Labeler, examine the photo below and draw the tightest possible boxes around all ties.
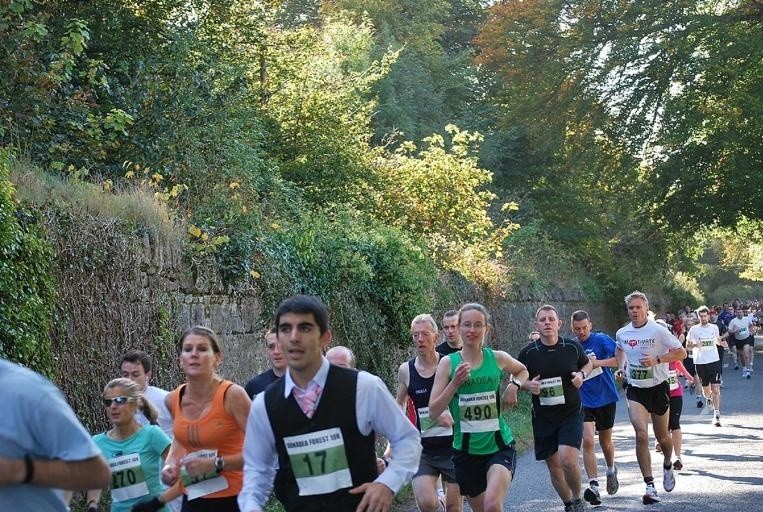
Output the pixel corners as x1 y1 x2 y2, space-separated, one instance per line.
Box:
291 385 322 417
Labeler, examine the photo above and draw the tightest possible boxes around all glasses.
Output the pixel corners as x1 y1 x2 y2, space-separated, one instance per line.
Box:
458 322 488 329
102 395 137 406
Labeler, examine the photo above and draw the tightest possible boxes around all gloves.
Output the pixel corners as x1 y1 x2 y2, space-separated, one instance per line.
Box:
128 496 165 512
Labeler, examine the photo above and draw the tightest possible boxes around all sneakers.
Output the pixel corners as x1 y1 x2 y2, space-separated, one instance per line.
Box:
714 416 721 427
662 462 676 492
573 499 585 512
607 466 619 496
642 485 661 506
673 459 684 471
683 361 757 409
583 486 602 506
563 501 573 512
